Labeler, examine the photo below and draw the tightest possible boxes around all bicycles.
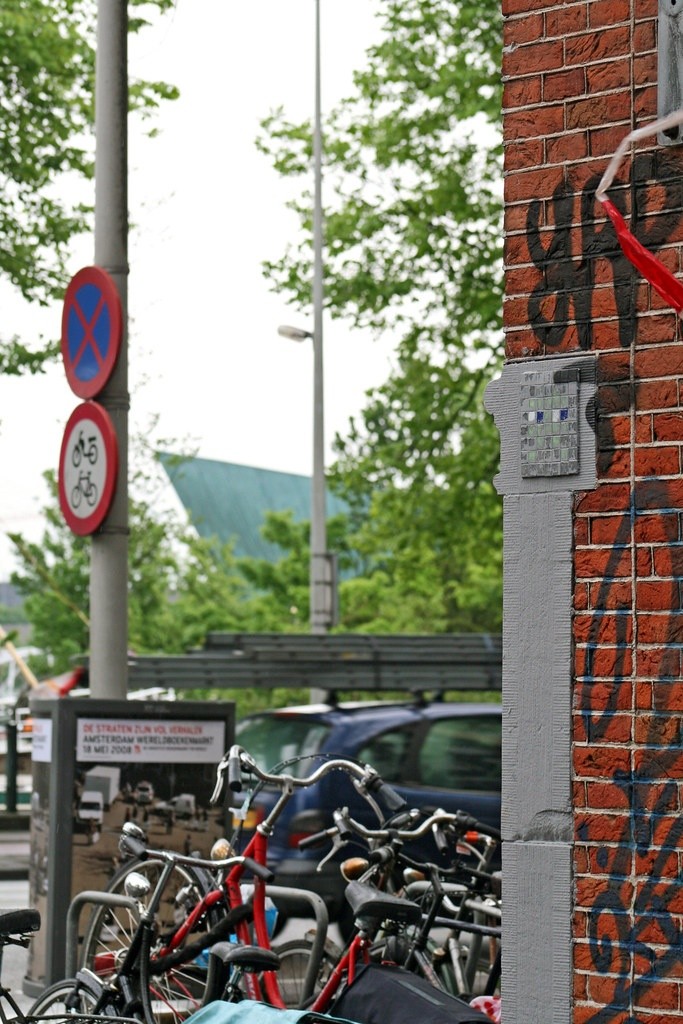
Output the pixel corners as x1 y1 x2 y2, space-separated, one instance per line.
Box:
0 743 501 1024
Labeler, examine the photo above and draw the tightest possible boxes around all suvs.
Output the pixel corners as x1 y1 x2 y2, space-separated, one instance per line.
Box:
235 700 502 904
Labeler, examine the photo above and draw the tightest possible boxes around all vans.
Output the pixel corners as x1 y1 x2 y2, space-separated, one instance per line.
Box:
76 790 103 833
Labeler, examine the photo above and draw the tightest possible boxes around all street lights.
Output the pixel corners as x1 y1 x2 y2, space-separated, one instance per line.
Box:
275 324 339 635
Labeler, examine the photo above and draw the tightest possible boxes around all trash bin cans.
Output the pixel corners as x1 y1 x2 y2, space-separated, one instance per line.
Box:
21 694 236 1000
